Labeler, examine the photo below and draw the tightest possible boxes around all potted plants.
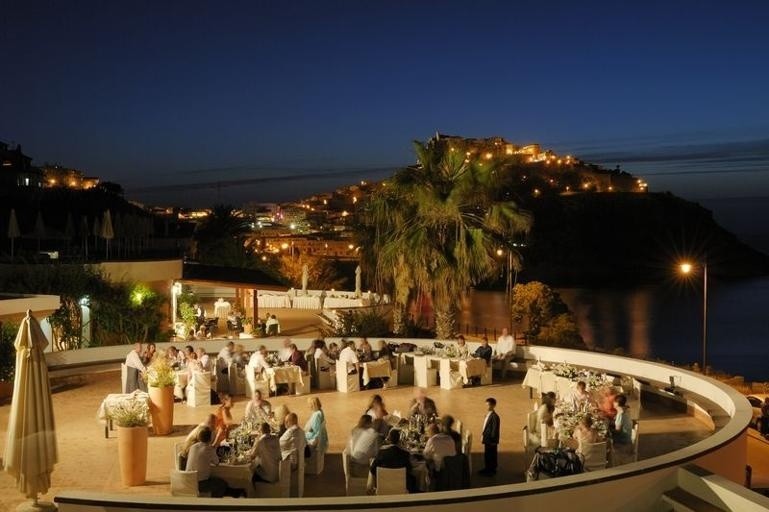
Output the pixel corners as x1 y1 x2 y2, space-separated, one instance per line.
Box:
104 395 155 487
138 354 179 435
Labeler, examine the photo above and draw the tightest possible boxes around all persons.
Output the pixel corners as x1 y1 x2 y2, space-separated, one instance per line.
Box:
124 299 638 499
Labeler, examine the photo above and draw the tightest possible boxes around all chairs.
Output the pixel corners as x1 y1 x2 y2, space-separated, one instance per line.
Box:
176 314 283 340
121 348 493 408
522 364 641 480
342 411 473 495
168 415 327 498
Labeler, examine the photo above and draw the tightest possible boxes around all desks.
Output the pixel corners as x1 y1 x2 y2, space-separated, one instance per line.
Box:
96 393 151 438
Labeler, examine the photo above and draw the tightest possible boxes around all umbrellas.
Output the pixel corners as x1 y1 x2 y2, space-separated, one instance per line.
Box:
0 306 60 506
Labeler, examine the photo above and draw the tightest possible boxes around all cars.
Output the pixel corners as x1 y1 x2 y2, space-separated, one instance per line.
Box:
745 392 769 431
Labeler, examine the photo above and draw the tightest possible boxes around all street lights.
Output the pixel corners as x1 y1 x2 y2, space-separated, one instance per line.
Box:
170 282 182 339
282 241 294 274
678 258 708 372
495 243 515 336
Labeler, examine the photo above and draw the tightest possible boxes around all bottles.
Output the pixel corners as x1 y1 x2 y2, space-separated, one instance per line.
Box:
224 412 255 457
556 393 587 419
564 361 566 366
399 412 439 451
